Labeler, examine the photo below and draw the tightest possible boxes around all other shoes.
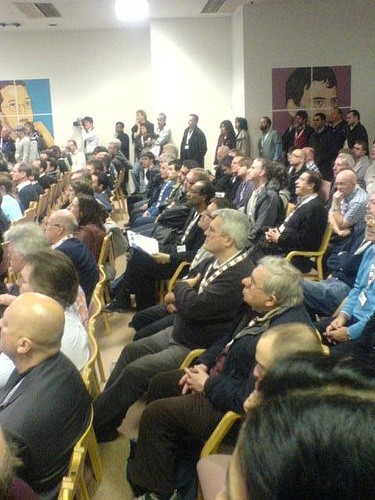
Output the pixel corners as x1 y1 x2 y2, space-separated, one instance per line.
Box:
134 488 180 500
100 292 131 312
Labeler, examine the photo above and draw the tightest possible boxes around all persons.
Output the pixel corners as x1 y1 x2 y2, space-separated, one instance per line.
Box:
135 122 160 157
114 121 130 161
78 116 100 157
286 110 314 150
257 116 283 163
180 113 207 168
14 125 33 164
131 109 155 142
328 106 350 140
344 110 369 155
23 121 45 151
308 113 337 180
0 126 16 163
150 112 172 145
234 116 251 157
1 140 375 500
213 119 237 165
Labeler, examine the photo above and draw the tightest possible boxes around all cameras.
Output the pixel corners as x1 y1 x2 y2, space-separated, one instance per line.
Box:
73 121 83 127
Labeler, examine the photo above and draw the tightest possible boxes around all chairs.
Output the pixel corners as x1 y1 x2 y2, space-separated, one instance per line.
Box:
85 289 100 339
94 263 111 337
66 401 105 500
159 174 339 460
2 166 127 262
81 330 102 408
94 229 115 305
